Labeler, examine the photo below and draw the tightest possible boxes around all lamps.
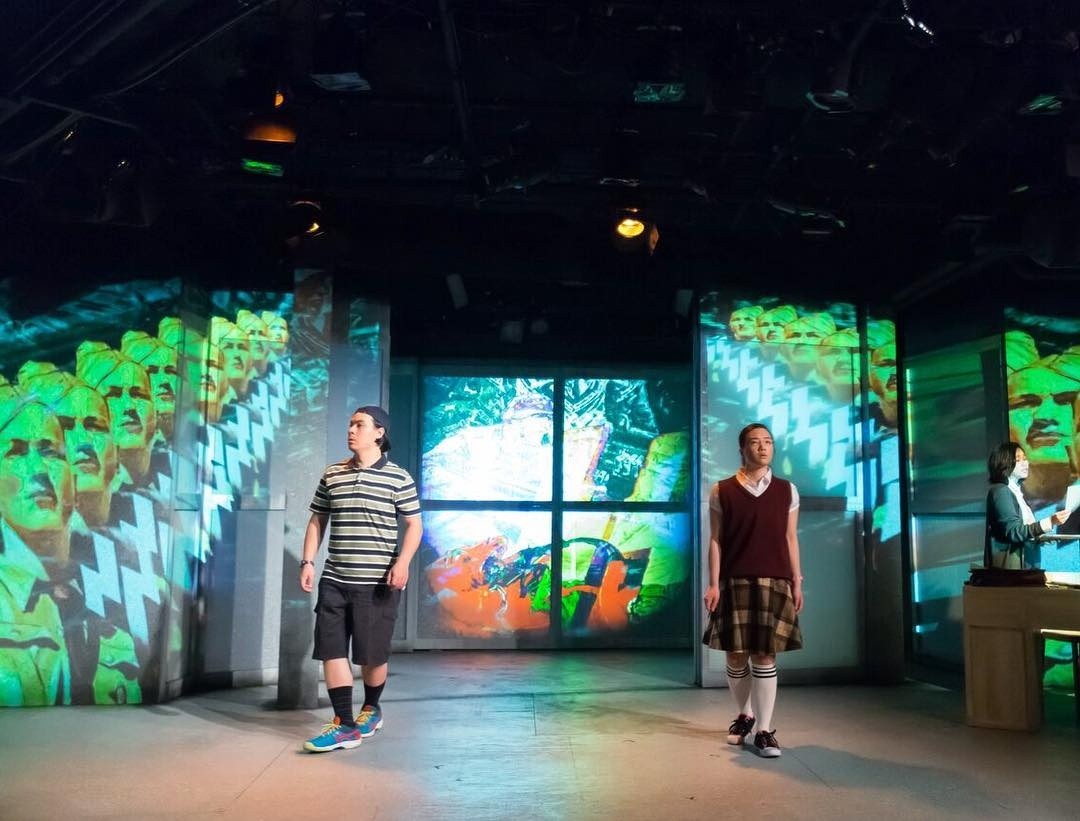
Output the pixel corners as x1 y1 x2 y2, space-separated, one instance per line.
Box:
616 206 645 241
286 200 328 241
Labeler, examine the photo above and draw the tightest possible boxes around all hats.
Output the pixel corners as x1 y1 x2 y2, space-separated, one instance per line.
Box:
357 405 393 453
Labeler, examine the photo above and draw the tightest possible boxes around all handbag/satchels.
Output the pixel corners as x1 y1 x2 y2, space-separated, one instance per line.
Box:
964 568 1050 589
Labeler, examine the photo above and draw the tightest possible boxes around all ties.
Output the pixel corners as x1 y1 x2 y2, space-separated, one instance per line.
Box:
35 580 94 706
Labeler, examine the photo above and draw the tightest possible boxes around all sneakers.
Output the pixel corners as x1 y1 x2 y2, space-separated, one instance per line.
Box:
302 714 362 753
753 731 781 757
353 703 383 737
727 712 757 745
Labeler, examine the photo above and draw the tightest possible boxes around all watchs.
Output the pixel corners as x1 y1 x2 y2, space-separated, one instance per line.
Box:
299 560 314 568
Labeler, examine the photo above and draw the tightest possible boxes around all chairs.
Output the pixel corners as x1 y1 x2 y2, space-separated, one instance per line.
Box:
1042 570 1080 719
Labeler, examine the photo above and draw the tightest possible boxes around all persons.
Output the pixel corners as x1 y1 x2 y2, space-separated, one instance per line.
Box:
696 295 1080 501
983 443 1073 570
1 310 288 708
704 423 804 756
298 406 425 752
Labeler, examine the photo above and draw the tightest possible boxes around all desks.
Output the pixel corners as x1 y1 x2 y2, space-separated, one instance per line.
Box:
963 582 1080 734
